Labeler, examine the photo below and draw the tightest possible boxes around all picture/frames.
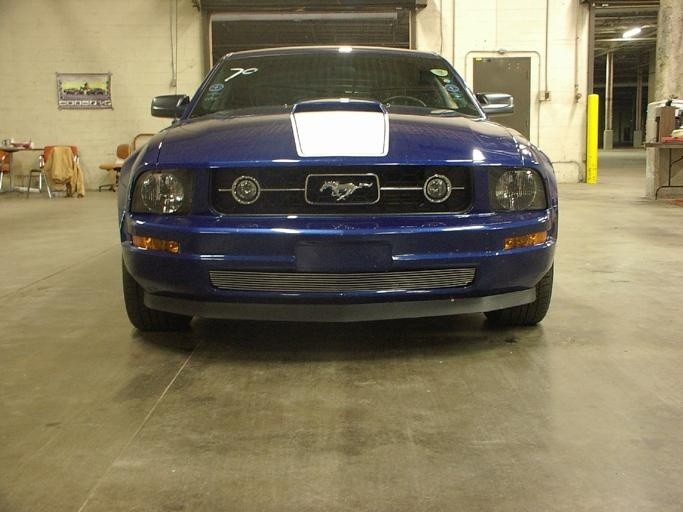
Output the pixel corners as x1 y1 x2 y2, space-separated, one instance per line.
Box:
55 71 113 110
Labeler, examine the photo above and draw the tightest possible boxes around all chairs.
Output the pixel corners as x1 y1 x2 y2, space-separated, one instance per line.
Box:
97 142 129 192
28 144 77 199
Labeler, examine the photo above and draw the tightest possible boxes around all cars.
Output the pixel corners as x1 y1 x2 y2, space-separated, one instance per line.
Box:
117 43 557 333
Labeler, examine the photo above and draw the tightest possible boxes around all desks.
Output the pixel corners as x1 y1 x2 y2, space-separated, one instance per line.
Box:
642 139 683 201
0 142 43 194
